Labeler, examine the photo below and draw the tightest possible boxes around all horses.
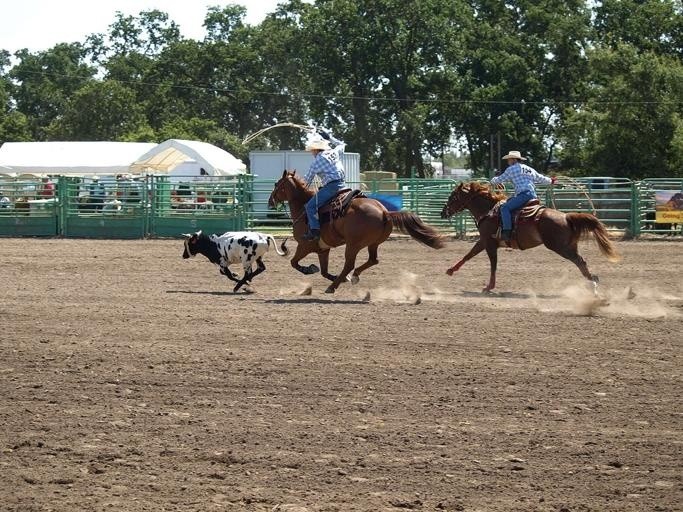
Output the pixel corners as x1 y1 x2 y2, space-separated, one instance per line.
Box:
440 181 616 299
267 169 446 293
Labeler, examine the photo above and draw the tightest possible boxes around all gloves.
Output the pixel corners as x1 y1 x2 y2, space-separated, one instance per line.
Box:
551 176 557 184
318 129 330 140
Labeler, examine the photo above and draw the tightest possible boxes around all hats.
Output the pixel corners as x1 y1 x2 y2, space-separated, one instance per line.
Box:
502 151 527 160
308 140 331 150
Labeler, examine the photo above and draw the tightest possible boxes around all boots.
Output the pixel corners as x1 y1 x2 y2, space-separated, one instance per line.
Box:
300 229 320 241
500 230 512 240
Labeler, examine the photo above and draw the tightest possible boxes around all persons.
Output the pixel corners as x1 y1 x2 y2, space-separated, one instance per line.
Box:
485 150 557 242
299 126 346 241
0 175 230 213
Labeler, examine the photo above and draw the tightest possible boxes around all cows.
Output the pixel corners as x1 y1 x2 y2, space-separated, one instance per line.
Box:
179 230 292 295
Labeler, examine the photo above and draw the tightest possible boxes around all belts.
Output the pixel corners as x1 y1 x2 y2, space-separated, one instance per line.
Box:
330 180 344 183
